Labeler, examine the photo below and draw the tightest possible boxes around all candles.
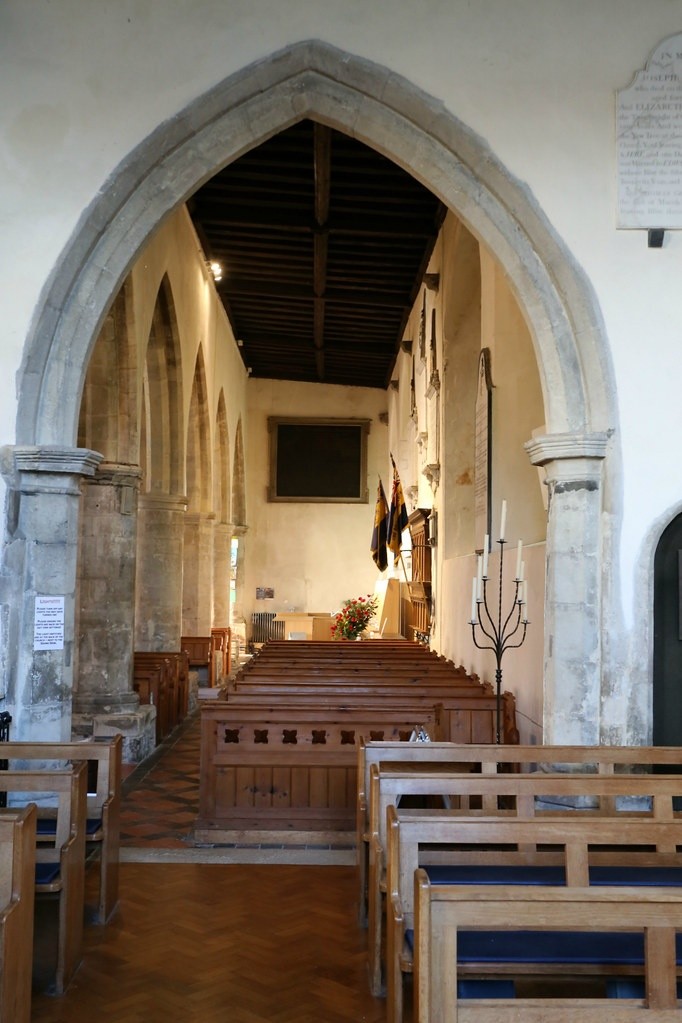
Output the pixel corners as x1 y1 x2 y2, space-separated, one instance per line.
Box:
483 532 490 576
516 538 522 578
477 556 481 602
499 498 507 539
518 561 525 600
523 580 528 620
472 575 477 621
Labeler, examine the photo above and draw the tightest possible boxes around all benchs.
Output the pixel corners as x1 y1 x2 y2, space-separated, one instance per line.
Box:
1 628 682 1023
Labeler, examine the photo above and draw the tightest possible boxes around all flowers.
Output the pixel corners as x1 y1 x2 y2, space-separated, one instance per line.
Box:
330 593 378 641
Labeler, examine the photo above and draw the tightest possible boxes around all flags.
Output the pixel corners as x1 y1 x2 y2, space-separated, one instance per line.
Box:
386 460 411 567
370 479 390 572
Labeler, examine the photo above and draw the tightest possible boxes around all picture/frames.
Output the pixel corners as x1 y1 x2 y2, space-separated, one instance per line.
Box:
266 415 370 505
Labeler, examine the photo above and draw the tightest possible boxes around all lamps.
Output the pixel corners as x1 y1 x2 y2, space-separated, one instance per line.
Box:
206 260 222 282
237 340 243 346
247 367 252 375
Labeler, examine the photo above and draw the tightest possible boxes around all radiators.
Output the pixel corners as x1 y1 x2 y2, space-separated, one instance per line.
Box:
252 613 286 640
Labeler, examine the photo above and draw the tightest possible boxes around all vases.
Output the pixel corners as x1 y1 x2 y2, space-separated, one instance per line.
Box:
348 622 364 642
